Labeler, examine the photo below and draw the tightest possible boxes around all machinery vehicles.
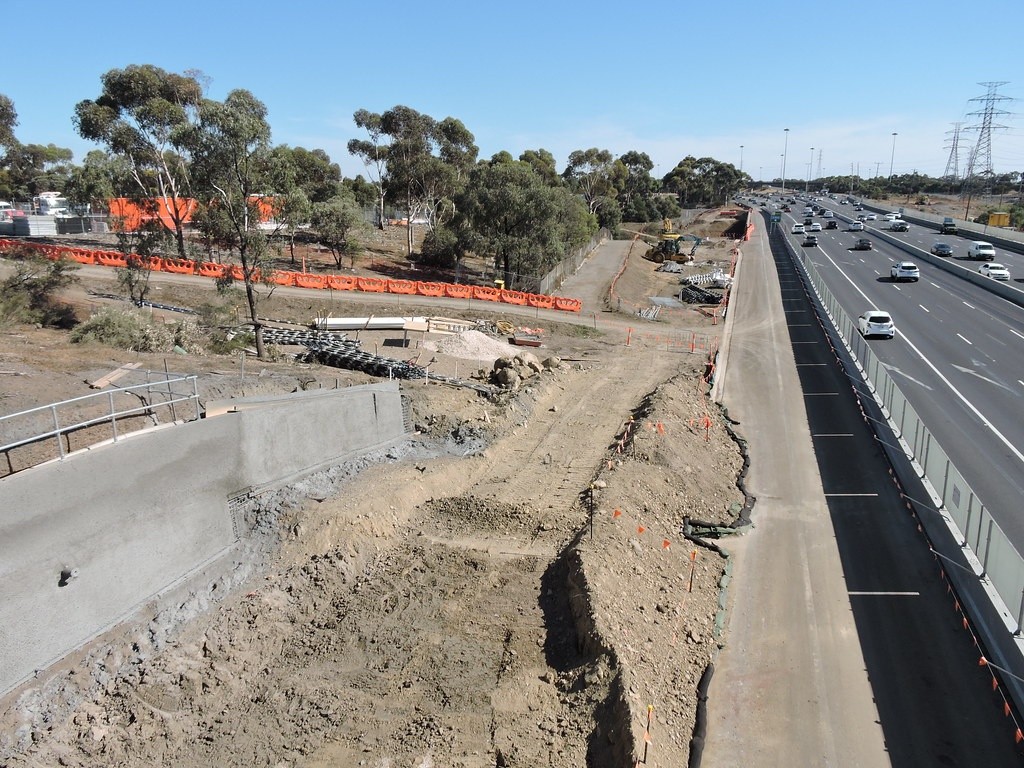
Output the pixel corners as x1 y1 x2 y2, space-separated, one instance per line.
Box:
645 217 702 265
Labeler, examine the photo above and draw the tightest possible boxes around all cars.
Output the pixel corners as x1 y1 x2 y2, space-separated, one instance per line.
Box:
931 242 953 257
855 239 872 250
858 310 895 339
979 262 1011 281
738 191 911 235
802 235 818 247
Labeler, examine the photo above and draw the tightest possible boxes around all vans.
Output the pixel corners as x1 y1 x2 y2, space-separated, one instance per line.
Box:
0 201 15 212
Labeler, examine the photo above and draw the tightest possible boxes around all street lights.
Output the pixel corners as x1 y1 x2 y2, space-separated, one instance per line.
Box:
889 132 898 184
805 163 812 197
740 145 743 173
782 129 790 192
809 148 815 181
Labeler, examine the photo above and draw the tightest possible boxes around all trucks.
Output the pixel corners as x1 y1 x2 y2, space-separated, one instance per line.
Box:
32 191 70 216
968 240 995 261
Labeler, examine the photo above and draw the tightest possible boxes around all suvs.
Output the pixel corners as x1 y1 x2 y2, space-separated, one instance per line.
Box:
891 262 919 282
941 222 959 235
